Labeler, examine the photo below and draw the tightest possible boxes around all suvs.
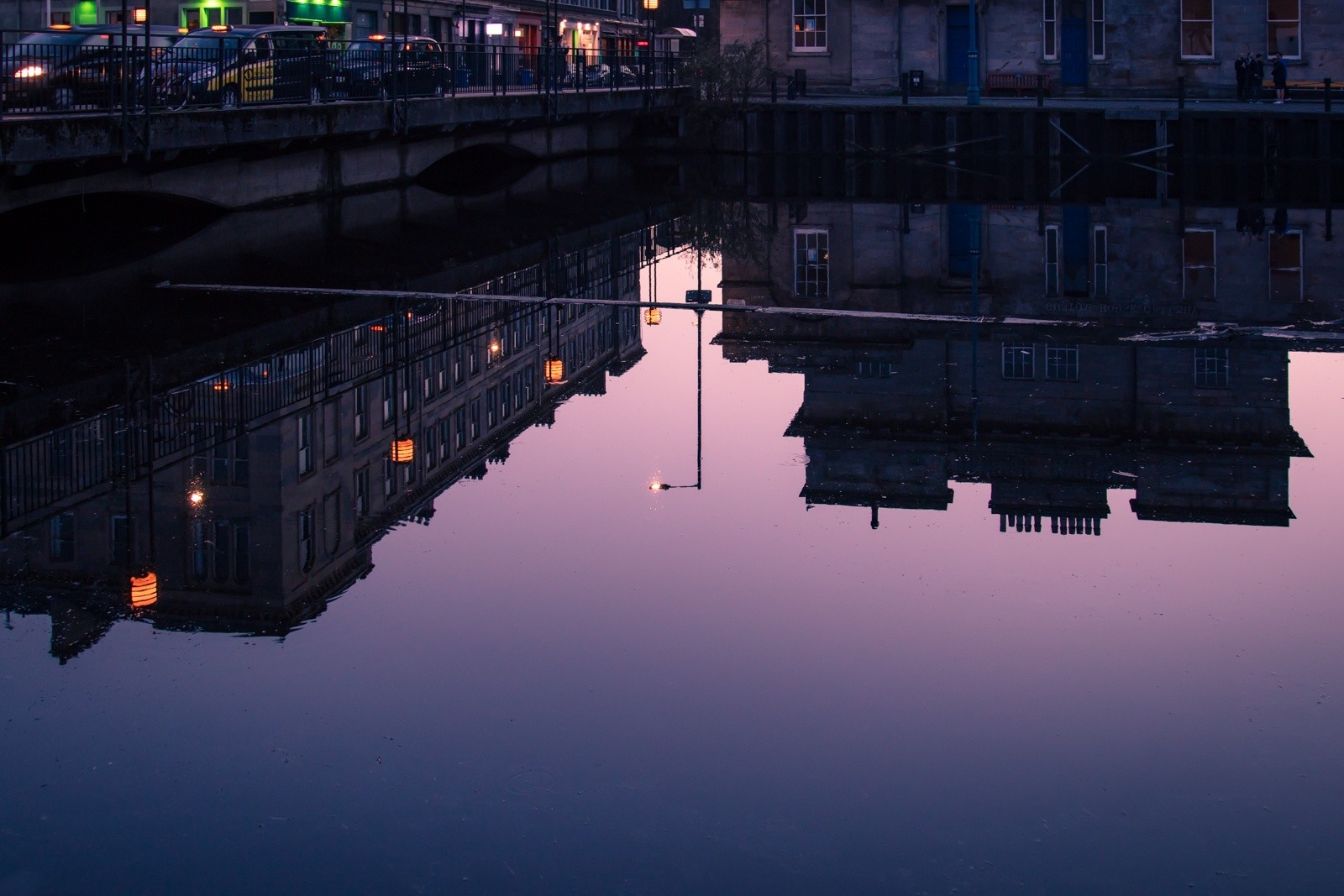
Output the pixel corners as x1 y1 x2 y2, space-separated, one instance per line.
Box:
0 24 190 110
329 33 448 99
135 24 329 109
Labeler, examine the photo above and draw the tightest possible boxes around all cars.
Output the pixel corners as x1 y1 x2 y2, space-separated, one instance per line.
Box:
572 64 676 88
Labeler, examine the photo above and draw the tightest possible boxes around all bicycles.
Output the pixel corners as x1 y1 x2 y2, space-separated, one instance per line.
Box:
125 48 193 115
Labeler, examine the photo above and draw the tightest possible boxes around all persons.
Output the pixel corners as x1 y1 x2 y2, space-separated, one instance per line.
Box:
1271 52 1292 104
538 36 568 93
307 32 336 103
416 41 435 60
1234 52 1265 104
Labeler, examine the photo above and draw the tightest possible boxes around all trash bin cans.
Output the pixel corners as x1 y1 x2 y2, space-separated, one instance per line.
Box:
458 69 471 87
522 69 535 86
794 68 806 97
909 70 923 97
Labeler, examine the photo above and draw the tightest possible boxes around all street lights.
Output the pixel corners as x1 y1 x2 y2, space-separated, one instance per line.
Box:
648 248 705 489
